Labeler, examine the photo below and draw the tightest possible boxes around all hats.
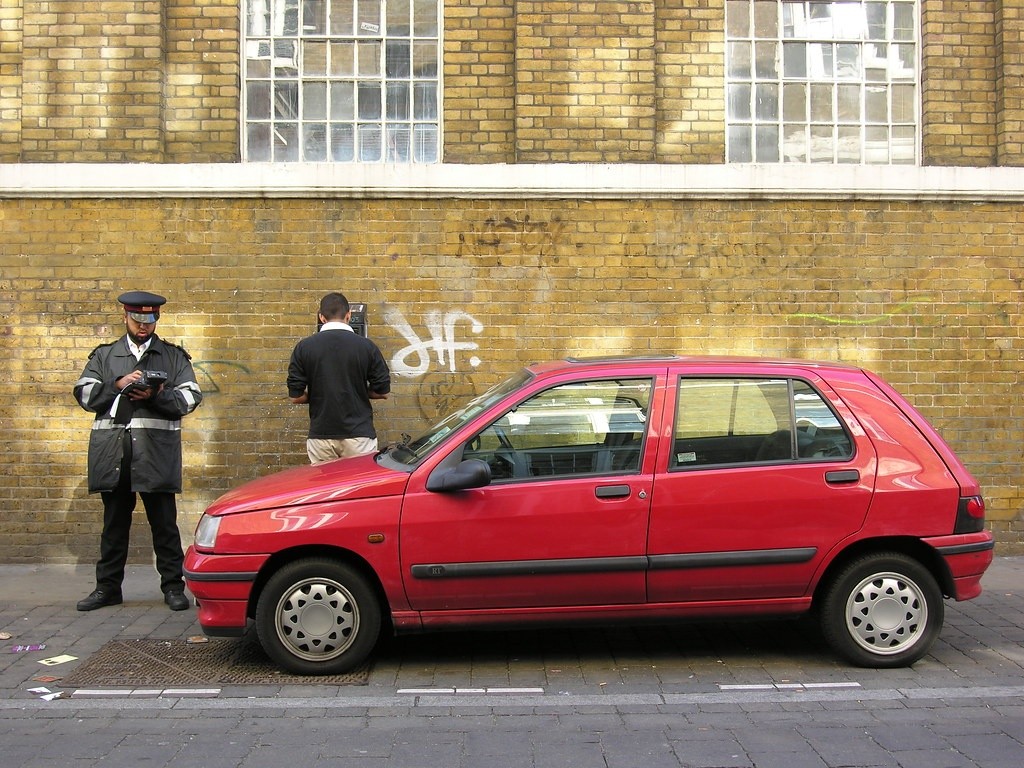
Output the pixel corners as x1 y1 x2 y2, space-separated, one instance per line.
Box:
117 290 168 325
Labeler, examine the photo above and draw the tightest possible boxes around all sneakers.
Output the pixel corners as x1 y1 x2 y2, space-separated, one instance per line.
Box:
163 588 189 611
76 588 123 611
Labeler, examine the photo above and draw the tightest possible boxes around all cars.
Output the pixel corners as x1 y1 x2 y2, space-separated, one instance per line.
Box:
181 354 996 677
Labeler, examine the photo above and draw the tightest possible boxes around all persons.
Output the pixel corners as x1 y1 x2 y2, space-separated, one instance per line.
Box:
73 291 203 610
287 293 391 464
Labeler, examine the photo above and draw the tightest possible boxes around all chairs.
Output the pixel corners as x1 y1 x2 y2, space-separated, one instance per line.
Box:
594 405 636 472
754 429 818 460
803 436 851 457
612 436 678 470
